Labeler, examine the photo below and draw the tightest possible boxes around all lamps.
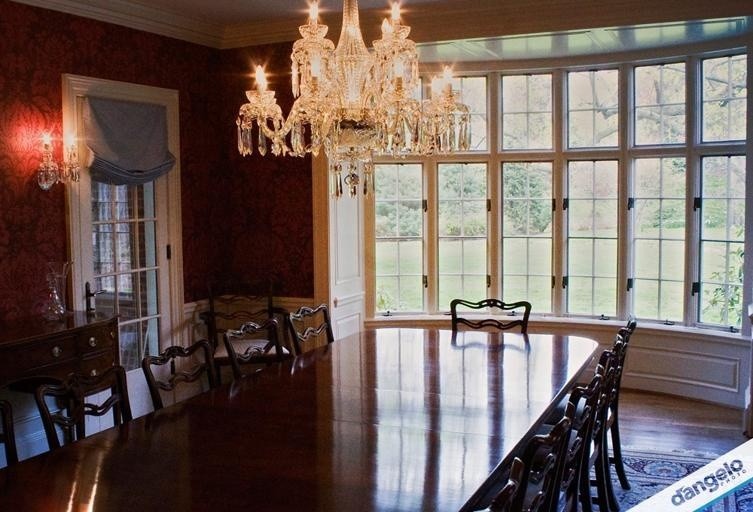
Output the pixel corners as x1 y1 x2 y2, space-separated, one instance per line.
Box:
233 0 469 198
37 132 81 189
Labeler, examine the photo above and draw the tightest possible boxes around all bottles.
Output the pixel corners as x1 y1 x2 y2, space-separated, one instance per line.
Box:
40 284 65 322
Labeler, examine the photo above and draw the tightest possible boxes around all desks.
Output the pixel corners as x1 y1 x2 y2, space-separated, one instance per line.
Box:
4 323 601 509
0 308 124 427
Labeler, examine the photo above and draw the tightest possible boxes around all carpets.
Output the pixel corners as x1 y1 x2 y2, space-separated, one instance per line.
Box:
563 440 753 512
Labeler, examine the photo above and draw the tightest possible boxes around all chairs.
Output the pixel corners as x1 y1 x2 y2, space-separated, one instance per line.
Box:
222 318 281 376
287 304 334 354
140 339 217 411
0 397 17 467
472 315 637 510
33 364 133 449
449 297 531 332
